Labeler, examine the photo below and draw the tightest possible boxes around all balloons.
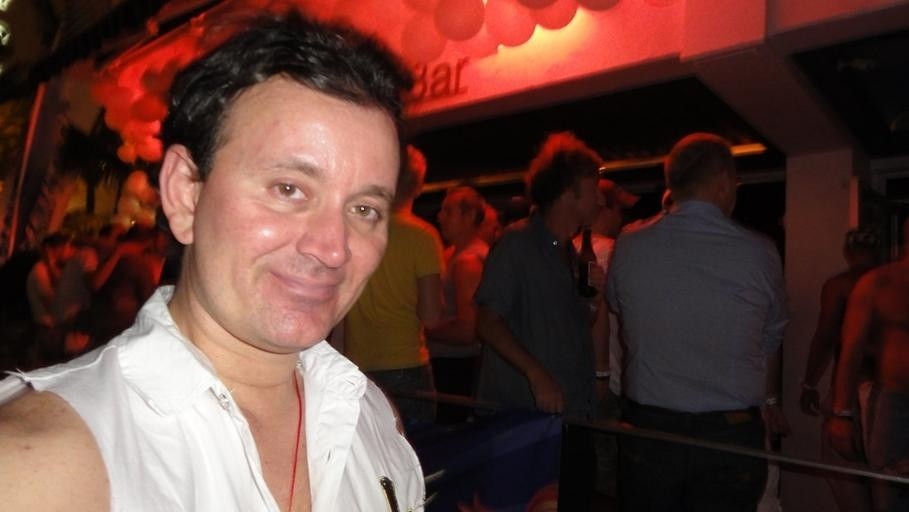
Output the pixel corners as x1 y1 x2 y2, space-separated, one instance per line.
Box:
55 2 623 237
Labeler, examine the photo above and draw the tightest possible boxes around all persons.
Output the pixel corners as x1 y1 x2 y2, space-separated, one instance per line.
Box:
832 220 907 510
799 228 883 510
1 123 793 511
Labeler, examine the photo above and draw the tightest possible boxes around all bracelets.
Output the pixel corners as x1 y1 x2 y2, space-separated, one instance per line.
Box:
801 383 819 392
832 407 856 419
